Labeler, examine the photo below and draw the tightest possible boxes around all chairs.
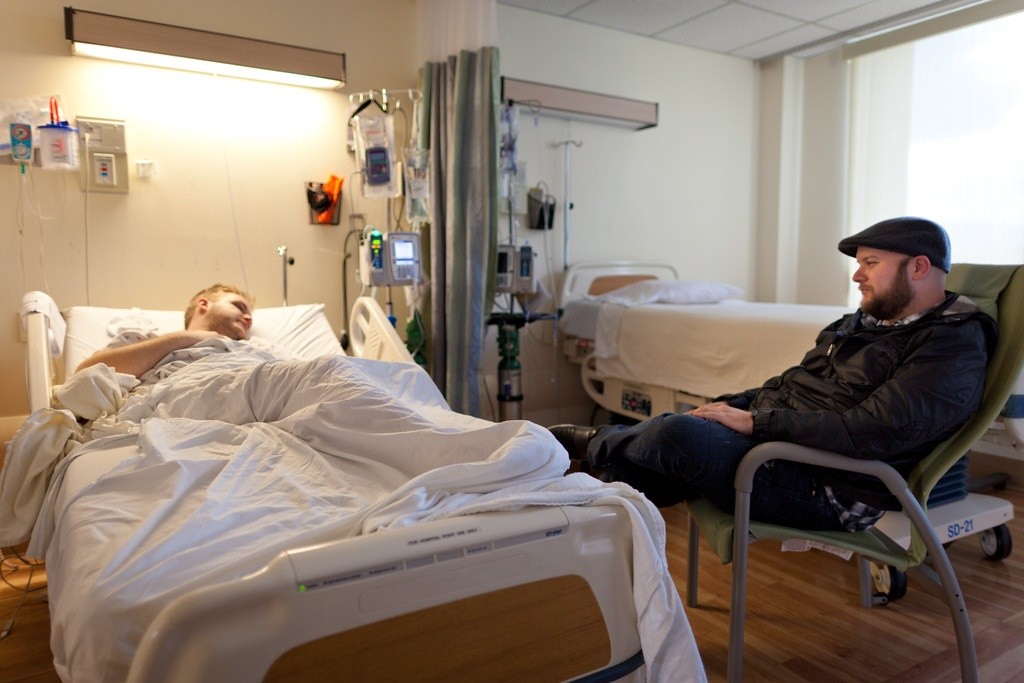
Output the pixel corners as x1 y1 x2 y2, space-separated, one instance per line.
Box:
685 264 1024 683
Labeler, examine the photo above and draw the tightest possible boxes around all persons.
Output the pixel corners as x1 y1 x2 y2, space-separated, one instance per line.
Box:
545 216 1003 533
72 282 257 377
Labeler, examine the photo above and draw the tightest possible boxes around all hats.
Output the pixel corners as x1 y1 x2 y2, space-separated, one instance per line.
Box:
837 217 951 274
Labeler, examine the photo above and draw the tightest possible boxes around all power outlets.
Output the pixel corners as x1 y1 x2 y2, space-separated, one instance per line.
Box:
136 161 155 178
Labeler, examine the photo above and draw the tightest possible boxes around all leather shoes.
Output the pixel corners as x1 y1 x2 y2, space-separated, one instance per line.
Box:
546 424 600 459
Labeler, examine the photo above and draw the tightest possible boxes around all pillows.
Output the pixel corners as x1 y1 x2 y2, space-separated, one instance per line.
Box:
624 279 725 304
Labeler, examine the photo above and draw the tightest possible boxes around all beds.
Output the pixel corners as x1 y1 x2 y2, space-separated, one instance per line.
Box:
19 291 668 683
558 260 1024 599
